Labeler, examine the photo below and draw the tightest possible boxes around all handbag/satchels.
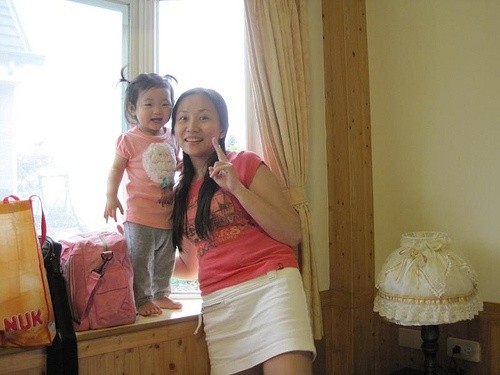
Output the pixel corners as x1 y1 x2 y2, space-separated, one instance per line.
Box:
0 195 56 347
59 225 136 332
38 235 62 277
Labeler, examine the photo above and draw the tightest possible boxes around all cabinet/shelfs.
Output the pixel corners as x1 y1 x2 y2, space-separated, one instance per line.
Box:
0 318 212 375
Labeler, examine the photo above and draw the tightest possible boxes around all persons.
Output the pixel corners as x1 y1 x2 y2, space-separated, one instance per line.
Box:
104 65 183 317
168 88 318 375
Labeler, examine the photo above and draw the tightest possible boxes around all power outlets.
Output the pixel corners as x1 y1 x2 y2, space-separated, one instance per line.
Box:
447 337 481 363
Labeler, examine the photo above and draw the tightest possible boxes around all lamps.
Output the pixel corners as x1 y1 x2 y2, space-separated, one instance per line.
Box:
372 230 483 375
